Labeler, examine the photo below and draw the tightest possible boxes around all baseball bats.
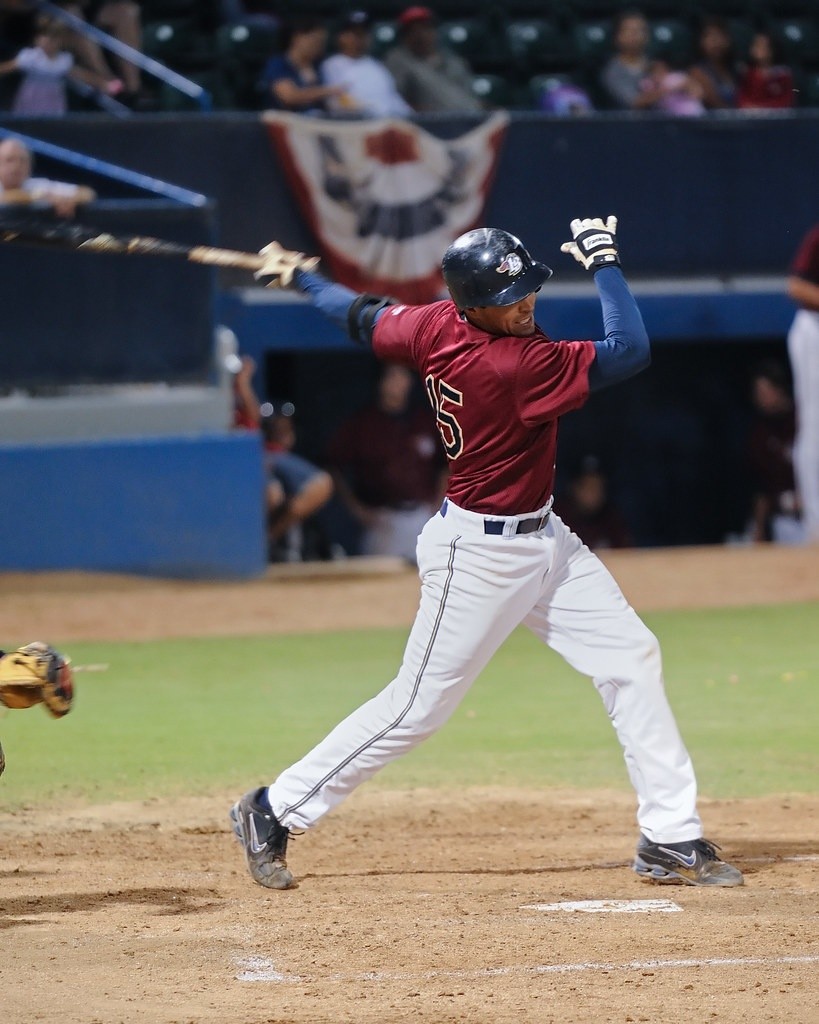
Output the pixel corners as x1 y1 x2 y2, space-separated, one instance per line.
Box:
0 220 329 274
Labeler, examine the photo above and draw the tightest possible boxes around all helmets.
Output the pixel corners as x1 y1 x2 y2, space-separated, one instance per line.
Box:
440 227 554 308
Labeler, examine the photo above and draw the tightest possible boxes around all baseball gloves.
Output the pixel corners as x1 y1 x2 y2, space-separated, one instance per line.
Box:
0 643 73 718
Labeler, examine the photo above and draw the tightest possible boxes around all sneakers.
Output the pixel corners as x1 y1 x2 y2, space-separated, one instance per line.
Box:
632 833 745 888
228 786 307 889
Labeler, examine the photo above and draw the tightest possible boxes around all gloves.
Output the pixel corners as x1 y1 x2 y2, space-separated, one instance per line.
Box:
251 241 322 291
562 215 622 275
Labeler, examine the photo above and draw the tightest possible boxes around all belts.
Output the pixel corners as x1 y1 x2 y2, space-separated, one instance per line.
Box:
438 502 551 536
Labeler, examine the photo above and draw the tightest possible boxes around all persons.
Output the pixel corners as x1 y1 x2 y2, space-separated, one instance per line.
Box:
234 356 452 571
0 642 73 778
0 0 144 118
742 366 802 543
0 137 97 220
233 216 748 891
785 224 819 540
250 8 796 117
551 463 637 551
